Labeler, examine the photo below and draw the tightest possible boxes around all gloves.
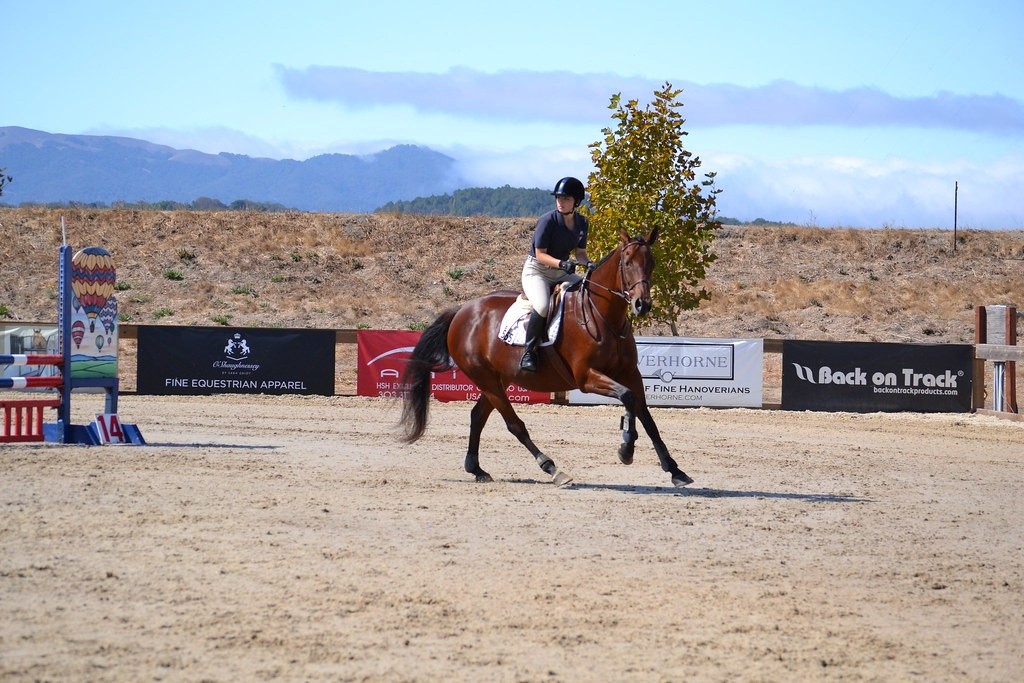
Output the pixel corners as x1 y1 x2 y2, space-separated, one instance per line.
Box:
558 260 576 275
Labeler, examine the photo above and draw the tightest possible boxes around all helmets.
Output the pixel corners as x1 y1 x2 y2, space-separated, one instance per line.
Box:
550 177 585 200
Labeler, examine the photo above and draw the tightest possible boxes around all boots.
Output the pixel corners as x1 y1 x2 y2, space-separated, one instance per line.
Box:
518 309 548 370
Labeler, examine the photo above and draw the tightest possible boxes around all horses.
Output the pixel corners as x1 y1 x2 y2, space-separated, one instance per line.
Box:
380 219 694 490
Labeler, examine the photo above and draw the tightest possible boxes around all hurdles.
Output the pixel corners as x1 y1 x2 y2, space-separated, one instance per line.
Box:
1 243 148 446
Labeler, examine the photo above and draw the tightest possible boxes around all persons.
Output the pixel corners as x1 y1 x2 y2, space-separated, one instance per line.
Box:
520 177 591 368
